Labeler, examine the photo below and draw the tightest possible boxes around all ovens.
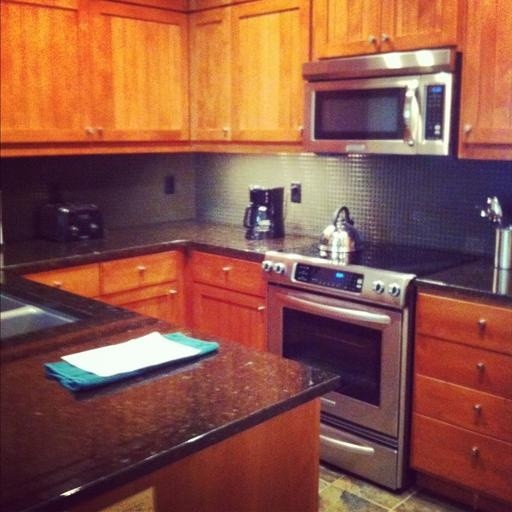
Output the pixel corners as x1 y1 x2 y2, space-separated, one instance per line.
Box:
265 283 411 494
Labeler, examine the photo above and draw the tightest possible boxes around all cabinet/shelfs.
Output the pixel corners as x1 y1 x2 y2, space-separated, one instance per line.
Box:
2 0 192 155
20 249 187 329
456 1 512 164
313 0 463 60
186 246 270 354
190 0 312 157
407 275 510 511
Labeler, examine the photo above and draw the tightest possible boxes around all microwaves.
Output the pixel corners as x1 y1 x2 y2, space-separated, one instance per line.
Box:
301 73 458 157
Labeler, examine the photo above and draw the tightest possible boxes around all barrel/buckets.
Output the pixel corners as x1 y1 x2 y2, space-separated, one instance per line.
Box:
492 224 512 270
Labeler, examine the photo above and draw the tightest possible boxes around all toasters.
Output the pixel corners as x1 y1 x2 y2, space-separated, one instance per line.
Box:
37 203 105 241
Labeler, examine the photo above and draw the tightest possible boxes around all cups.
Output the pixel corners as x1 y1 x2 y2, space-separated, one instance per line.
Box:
494 227 512 270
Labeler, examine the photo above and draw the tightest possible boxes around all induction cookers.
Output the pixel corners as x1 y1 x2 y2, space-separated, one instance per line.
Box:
261 241 481 309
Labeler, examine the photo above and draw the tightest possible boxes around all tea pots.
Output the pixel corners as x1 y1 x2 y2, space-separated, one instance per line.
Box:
314 205 365 252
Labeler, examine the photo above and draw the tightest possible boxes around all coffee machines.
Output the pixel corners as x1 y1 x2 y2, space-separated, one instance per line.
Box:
242 184 285 239
